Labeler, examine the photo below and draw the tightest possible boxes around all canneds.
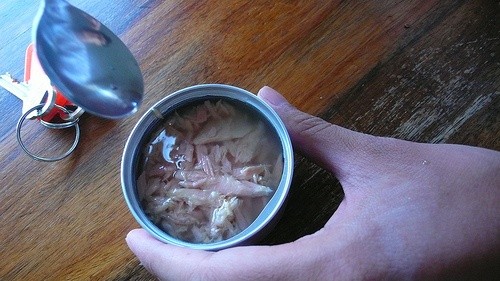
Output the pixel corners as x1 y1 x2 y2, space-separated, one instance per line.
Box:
121 83 295 251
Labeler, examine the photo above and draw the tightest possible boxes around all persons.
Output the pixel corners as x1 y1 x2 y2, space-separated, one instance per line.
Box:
123 84 500 280
